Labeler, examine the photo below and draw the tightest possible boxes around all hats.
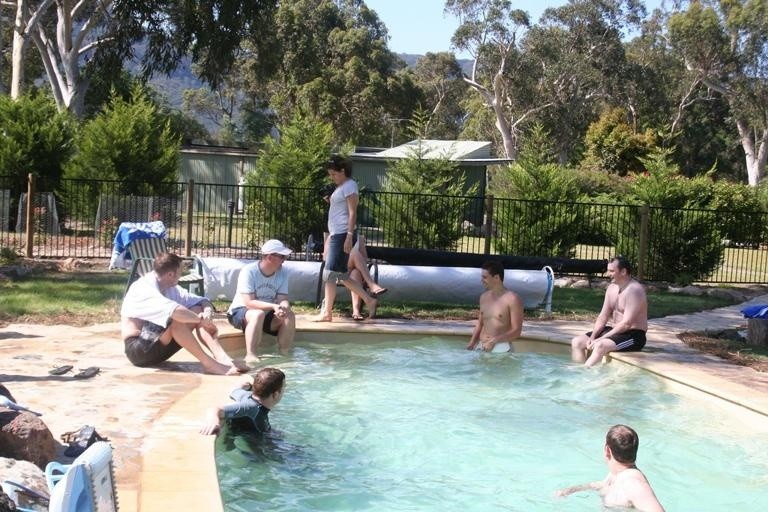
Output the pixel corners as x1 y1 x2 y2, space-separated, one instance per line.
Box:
261 239 293 256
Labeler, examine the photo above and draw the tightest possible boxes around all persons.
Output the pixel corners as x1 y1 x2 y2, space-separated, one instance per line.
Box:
553 424 666 512
570 257 648 367
120 252 250 377
323 229 388 321
465 259 524 352
307 156 379 326
198 367 314 479
225 239 296 363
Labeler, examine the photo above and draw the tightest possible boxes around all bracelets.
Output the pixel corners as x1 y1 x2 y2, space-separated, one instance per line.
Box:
347 231 353 234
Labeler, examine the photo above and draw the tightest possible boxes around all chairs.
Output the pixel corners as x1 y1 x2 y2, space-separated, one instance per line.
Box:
119 221 204 301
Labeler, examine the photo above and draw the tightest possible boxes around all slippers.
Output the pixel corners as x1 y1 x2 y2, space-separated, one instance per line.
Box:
351 314 364 321
372 288 388 296
